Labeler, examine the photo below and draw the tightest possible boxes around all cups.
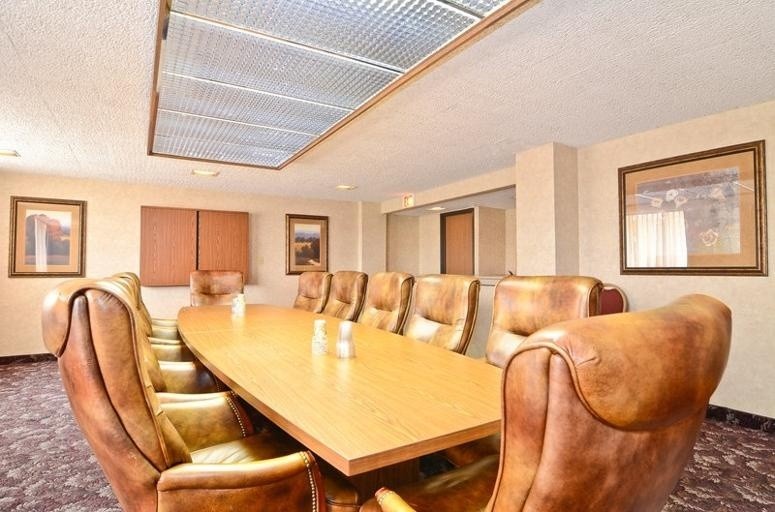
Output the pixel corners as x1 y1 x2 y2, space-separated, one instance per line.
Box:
310 319 329 357
335 320 356 359
232 293 246 314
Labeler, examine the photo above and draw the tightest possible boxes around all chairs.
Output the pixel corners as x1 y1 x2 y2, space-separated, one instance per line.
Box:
40 271 358 512
357 271 415 335
320 272 368 322
482 274 629 370
402 273 480 356
357 293 732 512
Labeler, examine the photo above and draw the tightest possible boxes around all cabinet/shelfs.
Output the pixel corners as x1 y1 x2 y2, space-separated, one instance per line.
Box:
139 205 250 288
440 208 475 276
176 304 503 477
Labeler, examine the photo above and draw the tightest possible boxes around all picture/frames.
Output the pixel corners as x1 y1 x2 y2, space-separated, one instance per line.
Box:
7 195 88 278
616 139 769 277
284 213 329 276
